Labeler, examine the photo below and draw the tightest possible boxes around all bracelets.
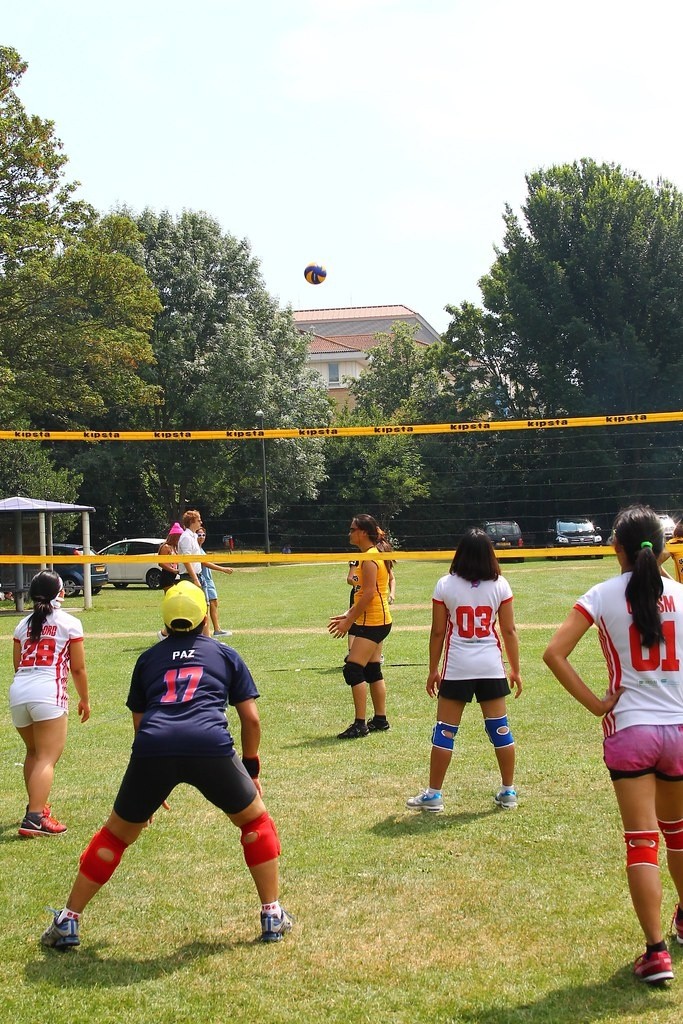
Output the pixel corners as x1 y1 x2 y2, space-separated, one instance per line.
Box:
242 755 261 779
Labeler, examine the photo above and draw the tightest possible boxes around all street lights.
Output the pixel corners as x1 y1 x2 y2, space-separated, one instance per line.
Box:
254 408 271 554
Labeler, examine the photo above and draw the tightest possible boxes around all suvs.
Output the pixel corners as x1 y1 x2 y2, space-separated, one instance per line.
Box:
47 543 110 598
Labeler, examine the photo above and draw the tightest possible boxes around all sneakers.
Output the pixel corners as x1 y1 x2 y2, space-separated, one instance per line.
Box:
670 903 683 944
26 804 52 817
633 940 674 983
405 790 444 813
260 908 295 943
337 723 371 739
366 717 389 732
494 790 516 809
18 813 68 837
39 908 80 948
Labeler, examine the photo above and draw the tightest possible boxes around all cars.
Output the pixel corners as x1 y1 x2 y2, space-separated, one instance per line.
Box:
97 537 166 591
658 515 677 541
548 518 604 561
479 519 525 563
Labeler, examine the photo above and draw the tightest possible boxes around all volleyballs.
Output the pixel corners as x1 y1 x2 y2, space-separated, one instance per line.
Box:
304 262 327 285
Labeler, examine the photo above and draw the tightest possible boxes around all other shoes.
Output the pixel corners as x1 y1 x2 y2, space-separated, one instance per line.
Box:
157 630 167 642
213 630 232 637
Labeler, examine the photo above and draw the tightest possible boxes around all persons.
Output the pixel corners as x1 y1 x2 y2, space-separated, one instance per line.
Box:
543 505 683 984
157 510 233 638
41 584 294 948
403 528 523 814
325 513 397 740
7 570 90 839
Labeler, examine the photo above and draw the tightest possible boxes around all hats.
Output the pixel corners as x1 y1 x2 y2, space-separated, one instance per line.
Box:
169 522 184 534
161 580 208 632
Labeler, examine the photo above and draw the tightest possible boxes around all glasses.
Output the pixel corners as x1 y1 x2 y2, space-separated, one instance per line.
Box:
349 528 360 534
197 519 201 522
197 533 206 538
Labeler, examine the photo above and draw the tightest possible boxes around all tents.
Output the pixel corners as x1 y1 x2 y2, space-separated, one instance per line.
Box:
0 495 96 609
